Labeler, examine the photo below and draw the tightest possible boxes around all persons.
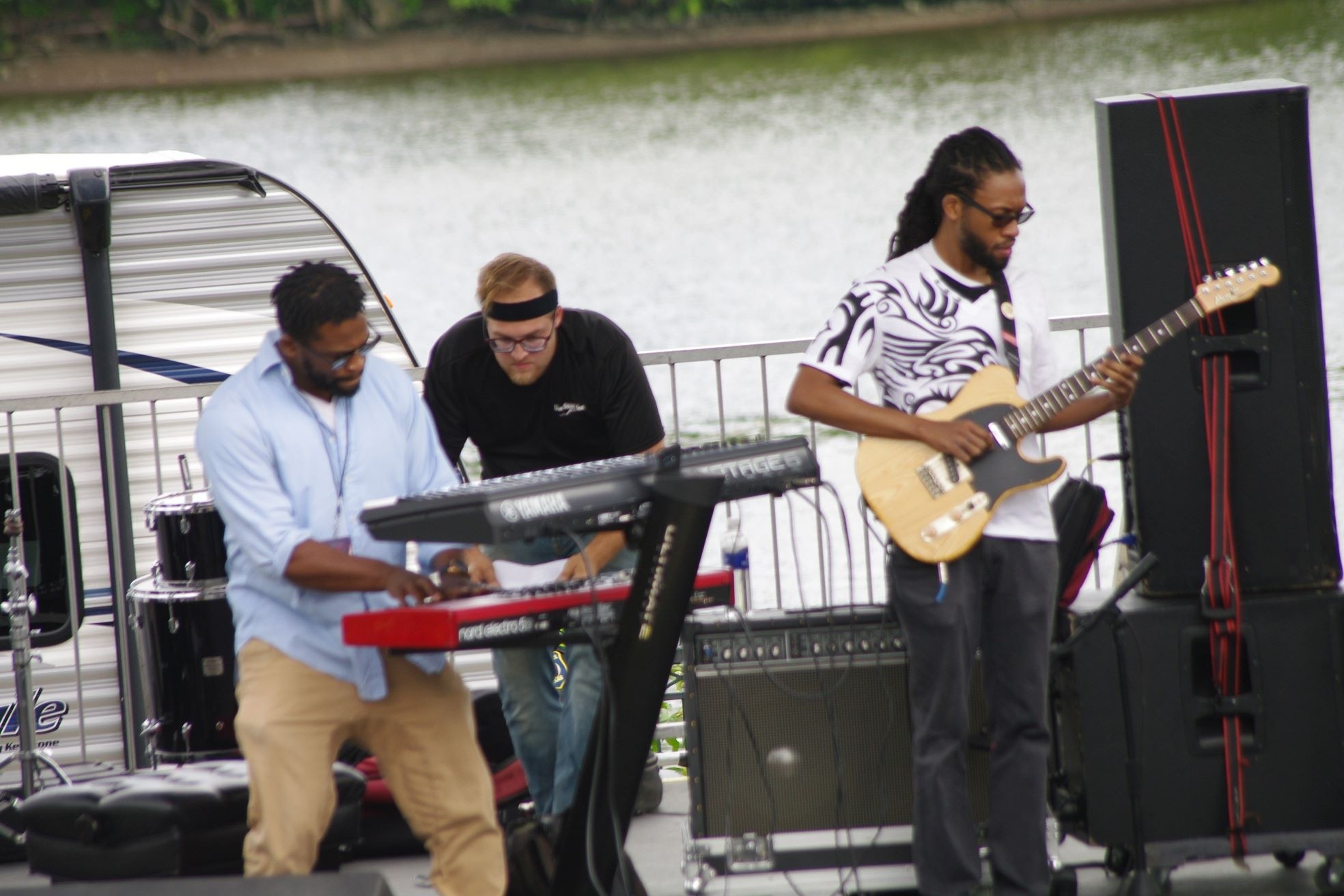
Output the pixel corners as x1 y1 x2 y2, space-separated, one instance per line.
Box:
786 124 1144 896
423 250 666 825
194 260 510 894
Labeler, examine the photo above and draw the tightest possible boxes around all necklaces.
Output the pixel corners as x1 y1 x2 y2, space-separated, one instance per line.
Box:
307 394 352 517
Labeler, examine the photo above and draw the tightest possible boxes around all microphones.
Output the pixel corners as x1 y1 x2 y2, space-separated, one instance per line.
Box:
1075 548 1160 637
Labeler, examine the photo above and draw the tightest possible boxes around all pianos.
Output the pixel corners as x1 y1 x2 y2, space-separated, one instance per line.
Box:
339 562 738 896
356 429 827 548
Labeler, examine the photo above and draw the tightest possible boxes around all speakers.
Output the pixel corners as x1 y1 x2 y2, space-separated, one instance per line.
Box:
1069 588 1344 860
685 618 914 846
1094 79 1344 591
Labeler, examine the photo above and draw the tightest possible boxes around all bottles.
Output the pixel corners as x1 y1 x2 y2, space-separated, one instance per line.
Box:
720 516 752 619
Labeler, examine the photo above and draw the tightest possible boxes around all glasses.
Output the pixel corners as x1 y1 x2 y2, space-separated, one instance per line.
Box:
483 307 556 353
952 191 1036 228
300 321 382 373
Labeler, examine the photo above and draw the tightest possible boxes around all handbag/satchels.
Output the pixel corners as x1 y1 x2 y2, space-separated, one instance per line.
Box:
1049 477 1116 609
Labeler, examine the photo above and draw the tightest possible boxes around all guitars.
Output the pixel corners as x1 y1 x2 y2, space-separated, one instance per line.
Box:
852 257 1282 566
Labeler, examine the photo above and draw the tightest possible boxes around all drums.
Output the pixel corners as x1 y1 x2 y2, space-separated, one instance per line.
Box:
142 485 230 590
124 569 245 766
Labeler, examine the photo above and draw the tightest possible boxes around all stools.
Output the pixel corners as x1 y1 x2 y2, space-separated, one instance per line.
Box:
12 759 368 886
